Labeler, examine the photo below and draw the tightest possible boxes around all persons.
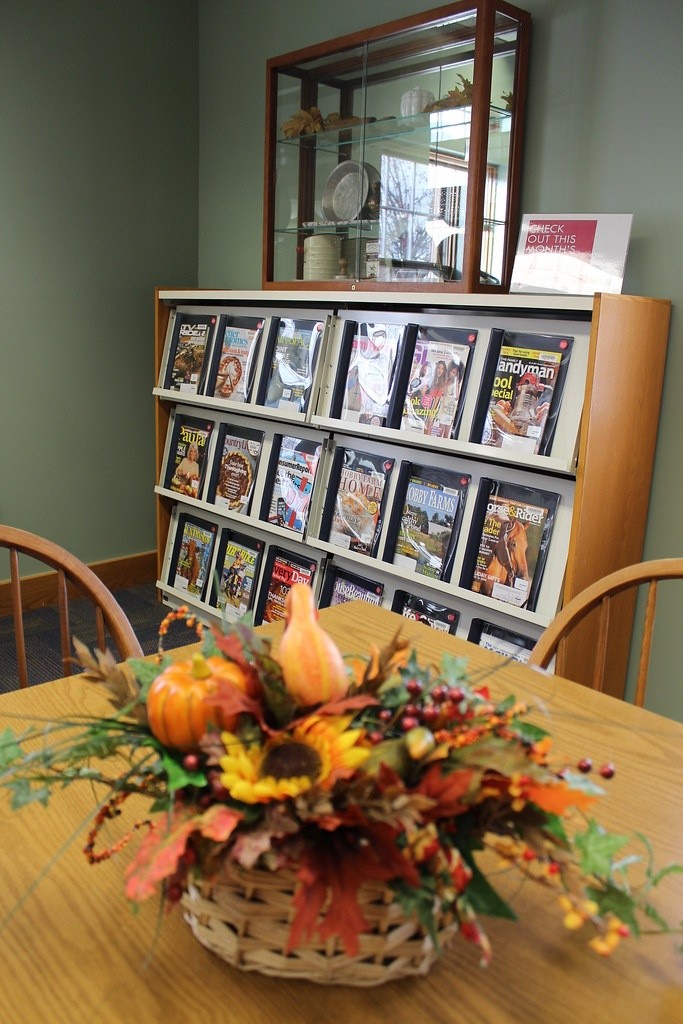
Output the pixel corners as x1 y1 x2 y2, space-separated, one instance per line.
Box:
422 359 465 439
224 550 247 597
173 440 200 487
516 379 533 396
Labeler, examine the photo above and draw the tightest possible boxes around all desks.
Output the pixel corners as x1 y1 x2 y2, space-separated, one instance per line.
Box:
0 598 683 1024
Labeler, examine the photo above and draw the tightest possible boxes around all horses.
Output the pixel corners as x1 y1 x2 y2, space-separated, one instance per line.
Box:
472 510 531 597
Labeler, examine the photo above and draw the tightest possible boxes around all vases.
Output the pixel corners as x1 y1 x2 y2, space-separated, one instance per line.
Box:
183 835 463 983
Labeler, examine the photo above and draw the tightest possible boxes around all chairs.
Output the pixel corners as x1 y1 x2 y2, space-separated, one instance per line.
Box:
528 557 683 708
0 524 146 688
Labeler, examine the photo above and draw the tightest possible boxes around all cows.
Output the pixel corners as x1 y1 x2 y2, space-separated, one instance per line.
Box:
180 540 200 584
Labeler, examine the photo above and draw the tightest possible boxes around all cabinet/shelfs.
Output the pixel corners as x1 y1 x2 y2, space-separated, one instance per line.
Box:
262 0 532 294
153 287 672 699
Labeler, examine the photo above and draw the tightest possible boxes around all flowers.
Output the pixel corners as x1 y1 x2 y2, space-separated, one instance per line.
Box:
0 583 683 974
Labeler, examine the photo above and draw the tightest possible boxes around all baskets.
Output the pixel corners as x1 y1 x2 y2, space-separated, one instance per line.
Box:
180 850 459 987
